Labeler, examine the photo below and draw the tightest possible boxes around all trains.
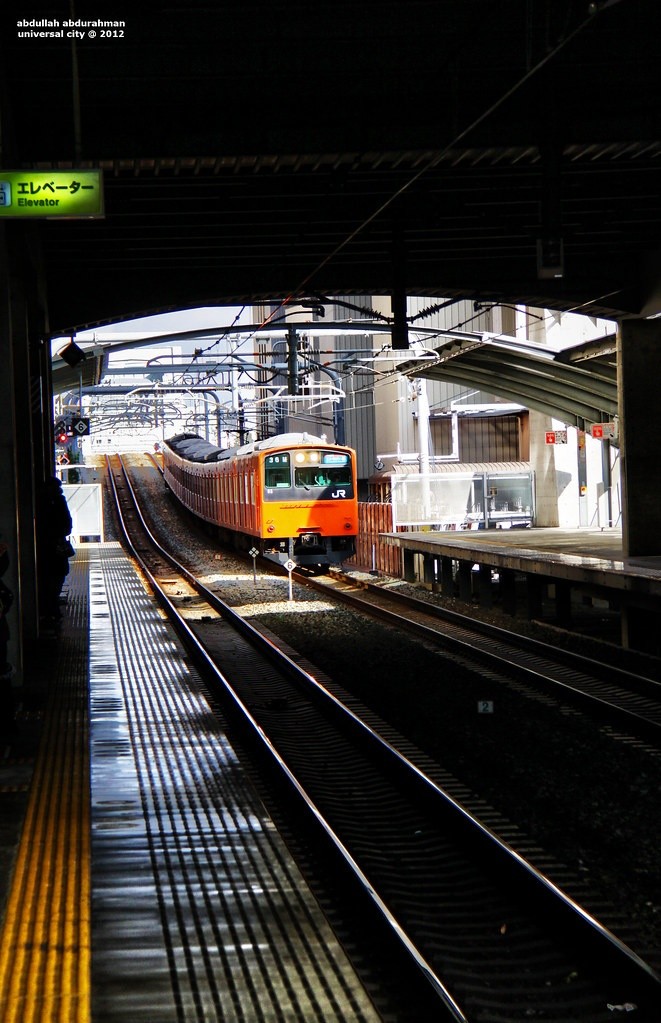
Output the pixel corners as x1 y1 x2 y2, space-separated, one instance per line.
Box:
162 432 358 575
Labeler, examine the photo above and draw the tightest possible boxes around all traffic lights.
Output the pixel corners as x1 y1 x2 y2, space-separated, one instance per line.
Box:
57 421 67 444
